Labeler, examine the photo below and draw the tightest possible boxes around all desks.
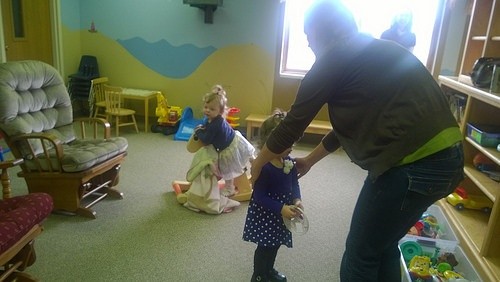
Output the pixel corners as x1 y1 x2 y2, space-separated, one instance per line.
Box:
122 87 160 134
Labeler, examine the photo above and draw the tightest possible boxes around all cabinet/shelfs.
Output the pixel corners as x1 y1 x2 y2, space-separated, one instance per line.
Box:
430 0 500 282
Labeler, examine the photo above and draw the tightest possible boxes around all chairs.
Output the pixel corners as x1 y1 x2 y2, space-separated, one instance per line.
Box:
67 55 102 118
105 91 139 137
0 157 54 282
0 60 130 219
92 77 119 126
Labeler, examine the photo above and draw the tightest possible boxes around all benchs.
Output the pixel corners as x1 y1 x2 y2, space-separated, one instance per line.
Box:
246 114 332 143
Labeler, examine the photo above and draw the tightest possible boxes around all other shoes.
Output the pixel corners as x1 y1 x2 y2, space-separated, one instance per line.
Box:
251 273 274 282
270 269 287 282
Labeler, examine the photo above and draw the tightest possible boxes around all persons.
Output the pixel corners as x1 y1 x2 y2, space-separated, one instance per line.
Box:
195 92 256 196
243 113 305 282
380 9 416 52
251 0 465 282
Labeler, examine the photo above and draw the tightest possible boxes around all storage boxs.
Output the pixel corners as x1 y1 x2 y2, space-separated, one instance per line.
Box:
465 122 500 147
398 238 484 282
399 204 460 251
